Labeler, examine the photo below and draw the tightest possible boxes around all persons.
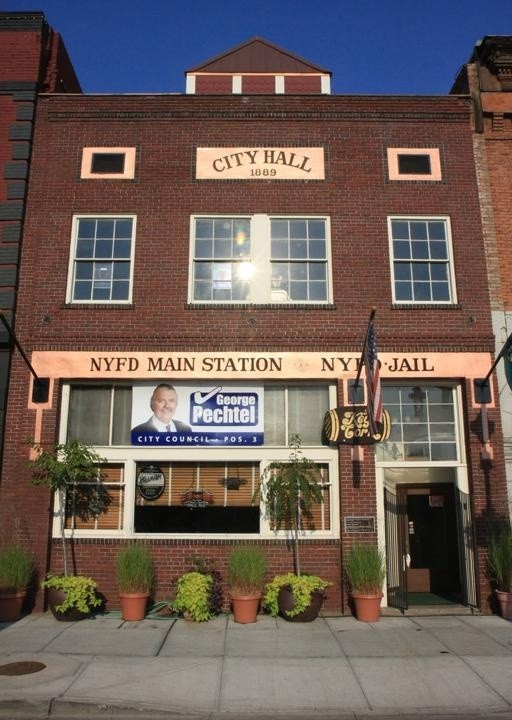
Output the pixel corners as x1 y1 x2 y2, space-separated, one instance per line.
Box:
129 383 194 436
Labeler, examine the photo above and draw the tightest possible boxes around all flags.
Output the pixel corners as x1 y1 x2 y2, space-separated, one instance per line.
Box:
362 318 385 438
499 332 511 392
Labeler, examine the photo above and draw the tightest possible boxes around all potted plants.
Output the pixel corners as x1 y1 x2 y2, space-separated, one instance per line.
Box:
474 510 512 619
